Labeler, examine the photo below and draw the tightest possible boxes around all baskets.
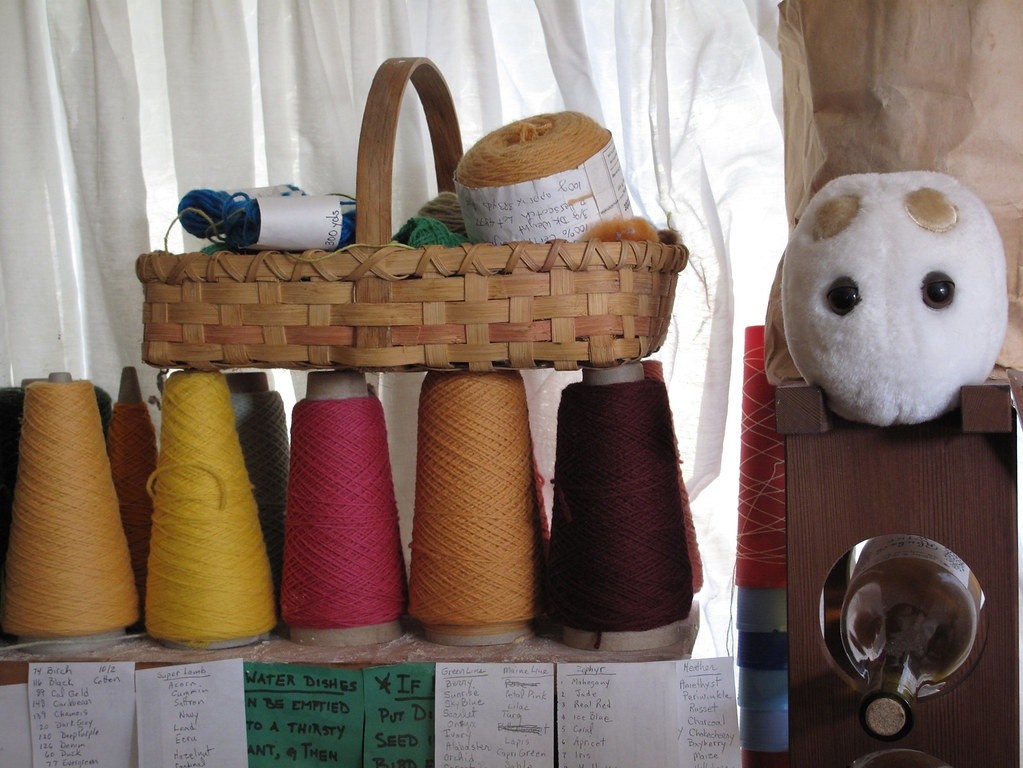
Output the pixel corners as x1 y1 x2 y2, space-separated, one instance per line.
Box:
137 57 690 375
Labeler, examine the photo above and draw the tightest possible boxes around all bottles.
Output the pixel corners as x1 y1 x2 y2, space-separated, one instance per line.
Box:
851 749 952 768
839 533 982 743
